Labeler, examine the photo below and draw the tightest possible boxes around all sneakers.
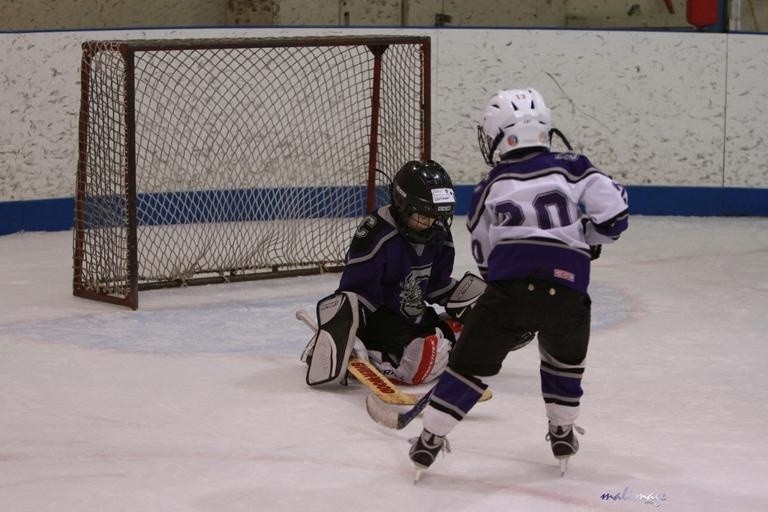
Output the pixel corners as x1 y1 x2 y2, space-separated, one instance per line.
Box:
549 420 579 457
408 430 444 467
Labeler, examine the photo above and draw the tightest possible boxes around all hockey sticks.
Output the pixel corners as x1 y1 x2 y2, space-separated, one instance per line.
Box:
296 310 493 404
366 387 437 429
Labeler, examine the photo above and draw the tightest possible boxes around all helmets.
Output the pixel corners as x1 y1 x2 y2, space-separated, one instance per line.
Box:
392 160 455 244
478 87 551 167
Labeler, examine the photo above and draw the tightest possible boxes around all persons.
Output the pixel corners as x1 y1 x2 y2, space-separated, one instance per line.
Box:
301 158 535 387
409 86 630 467
